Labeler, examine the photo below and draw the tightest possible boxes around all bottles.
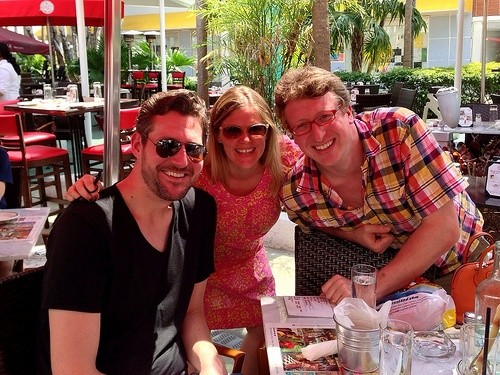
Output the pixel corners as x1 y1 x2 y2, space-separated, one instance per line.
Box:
459 310 483 357
474 241 500 375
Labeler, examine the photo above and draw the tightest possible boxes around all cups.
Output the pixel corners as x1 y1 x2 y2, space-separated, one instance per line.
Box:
379 319 412 375
332 311 379 373
67 84 79 104
488 105 498 127
463 324 496 375
351 264 376 310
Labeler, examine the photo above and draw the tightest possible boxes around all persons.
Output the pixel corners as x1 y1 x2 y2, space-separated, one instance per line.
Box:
273 68 490 307
64 86 303 375
0 42 21 101
43 88 229 375
0 147 14 277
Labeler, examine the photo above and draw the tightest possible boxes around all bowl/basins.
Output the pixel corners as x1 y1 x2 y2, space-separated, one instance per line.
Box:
411 331 456 361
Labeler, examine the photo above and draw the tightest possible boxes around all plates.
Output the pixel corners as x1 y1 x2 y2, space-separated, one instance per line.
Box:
0 211 20 224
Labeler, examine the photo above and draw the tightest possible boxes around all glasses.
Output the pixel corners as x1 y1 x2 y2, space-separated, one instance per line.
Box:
220 122 269 139
289 105 342 136
140 131 208 163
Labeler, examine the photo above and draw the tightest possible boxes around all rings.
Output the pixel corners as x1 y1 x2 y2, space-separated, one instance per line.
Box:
330 297 336 303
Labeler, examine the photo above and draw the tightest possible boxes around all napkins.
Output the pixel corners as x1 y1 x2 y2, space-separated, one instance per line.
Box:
302 339 339 361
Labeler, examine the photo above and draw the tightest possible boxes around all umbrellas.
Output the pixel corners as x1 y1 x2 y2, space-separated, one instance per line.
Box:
0 27 54 55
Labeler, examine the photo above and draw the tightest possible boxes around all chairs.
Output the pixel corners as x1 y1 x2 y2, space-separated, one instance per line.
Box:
79 102 143 182
0 97 57 183
294 223 437 296
356 93 392 114
52 81 88 165
462 102 491 121
391 82 403 105
0 266 247 375
121 71 145 98
353 85 380 94
396 88 418 110
166 71 186 90
0 114 74 231
141 70 161 98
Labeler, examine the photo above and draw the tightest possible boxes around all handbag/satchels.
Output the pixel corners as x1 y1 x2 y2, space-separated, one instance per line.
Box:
451 232 495 324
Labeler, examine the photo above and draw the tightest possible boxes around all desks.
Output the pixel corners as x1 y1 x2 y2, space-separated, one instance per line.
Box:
465 175 500 214
260 294 500 375
426 118 500 159
4 97 141 175
0 207 50 272
